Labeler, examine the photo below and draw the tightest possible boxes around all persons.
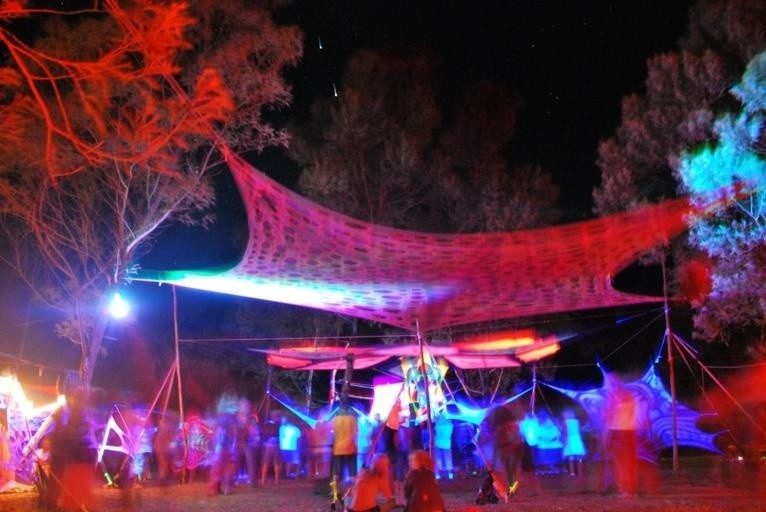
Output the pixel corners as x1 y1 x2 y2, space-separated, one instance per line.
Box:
31 437 52 479
153 373 644 511
